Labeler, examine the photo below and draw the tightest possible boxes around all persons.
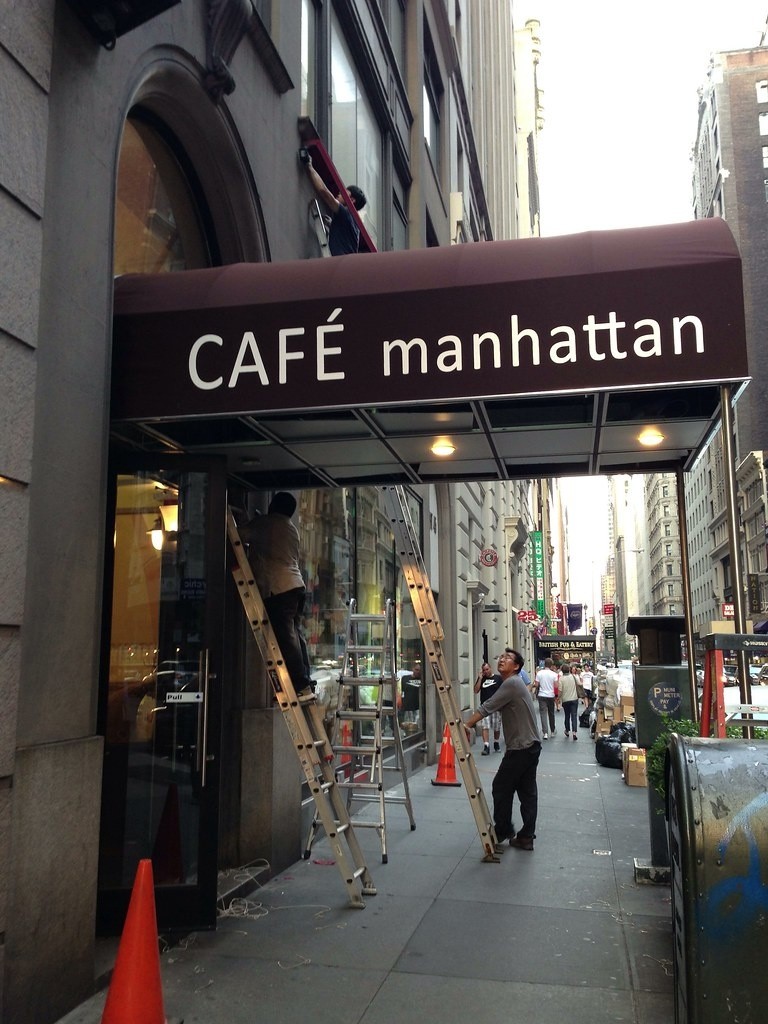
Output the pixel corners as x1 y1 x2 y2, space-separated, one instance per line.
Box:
462 646 544 851
381 661 422 737
533 658 559 740
550 663 594 712
240 490 318 702
473 663 503 755
518 669 532 691
558 665 582 741
303 157 367 255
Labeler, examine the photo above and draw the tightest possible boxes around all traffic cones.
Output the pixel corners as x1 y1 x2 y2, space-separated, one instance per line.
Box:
97 856 178 1024
430 722 462 788
341 722 355 767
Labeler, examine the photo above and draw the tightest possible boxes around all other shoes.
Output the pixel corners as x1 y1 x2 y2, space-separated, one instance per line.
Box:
491 827 516 844
543 735 548 738
297 690 315 702
481 745 490 756
573 735 578 740
551 729 557 738
557 708 560 711
509 832 533 850
564 730 569 736
493 742 502 753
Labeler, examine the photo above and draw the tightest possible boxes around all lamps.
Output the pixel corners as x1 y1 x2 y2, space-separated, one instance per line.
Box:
159 505 178 539
145 518 163 550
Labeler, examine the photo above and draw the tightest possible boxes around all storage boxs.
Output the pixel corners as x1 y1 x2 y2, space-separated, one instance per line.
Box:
594 667 635 734
625 747 647 786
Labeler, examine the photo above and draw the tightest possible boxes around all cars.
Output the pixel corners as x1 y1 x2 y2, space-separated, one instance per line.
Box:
725 664 740 687
736 663 768 686
695 664 728 687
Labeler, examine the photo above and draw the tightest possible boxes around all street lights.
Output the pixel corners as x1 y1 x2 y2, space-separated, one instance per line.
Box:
608 548 647 603
583 603 589 636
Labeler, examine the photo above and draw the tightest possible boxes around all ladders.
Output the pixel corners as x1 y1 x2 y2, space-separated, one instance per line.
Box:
379 484 504 866
304 598 417 864
225 505 379 907
700 633 768 738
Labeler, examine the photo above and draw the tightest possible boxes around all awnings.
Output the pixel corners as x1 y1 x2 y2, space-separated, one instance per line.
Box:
113 217 754 740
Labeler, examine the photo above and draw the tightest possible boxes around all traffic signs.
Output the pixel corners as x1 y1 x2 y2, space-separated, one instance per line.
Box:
604 630 615 635
605 635 614 639
604 627 614 631
604 604 615 614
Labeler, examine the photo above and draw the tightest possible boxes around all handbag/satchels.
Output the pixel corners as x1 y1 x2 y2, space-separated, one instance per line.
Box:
573 674 585 698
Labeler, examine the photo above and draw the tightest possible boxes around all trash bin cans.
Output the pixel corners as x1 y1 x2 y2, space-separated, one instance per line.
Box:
663 732 767 1024
